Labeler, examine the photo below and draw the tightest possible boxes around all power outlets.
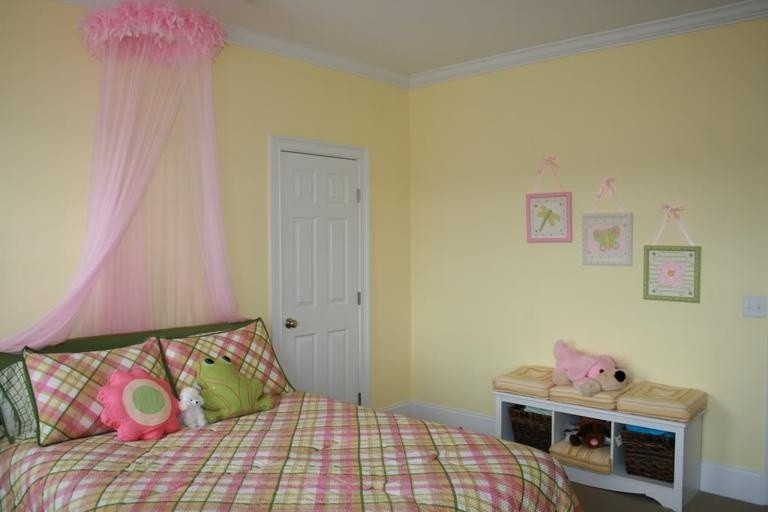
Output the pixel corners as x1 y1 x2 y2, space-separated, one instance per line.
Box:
742 295 766 317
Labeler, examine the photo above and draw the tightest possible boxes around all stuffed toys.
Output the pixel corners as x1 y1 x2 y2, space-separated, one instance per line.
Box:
175 386 207 429
551 339 634 397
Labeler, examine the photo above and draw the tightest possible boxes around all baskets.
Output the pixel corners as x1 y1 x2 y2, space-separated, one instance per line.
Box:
507 403 552 454
619 425 675 484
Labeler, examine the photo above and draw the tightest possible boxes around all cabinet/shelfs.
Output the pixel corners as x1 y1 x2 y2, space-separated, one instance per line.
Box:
494 392 702 512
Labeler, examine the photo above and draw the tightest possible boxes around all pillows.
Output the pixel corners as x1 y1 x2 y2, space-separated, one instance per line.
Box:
1 315 294 448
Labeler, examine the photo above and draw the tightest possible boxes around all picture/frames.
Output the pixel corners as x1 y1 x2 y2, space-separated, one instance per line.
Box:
527 194 701 304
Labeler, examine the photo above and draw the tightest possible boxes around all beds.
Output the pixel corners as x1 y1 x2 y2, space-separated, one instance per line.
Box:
0 320 557 512
